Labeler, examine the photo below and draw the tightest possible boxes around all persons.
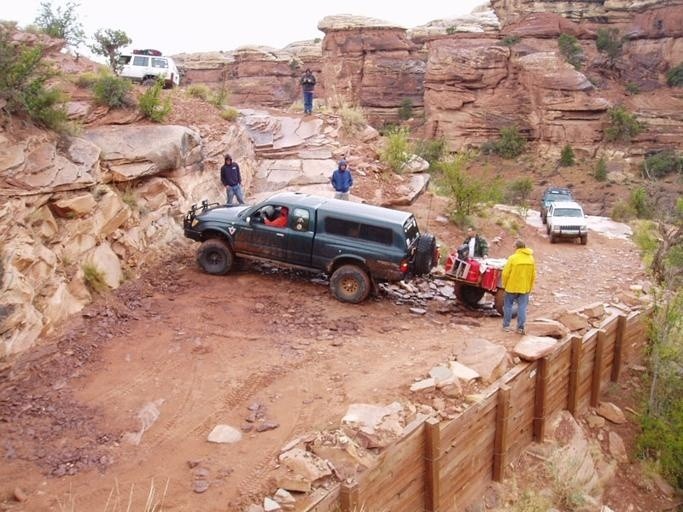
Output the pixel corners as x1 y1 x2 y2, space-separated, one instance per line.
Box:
263 207 289 228
331 160 355 203
463 225 488 305
220 154 245 206
300 67 316 116
501 240 538 336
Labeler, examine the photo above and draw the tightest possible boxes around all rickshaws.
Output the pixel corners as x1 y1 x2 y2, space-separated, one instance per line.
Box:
430 255 528 318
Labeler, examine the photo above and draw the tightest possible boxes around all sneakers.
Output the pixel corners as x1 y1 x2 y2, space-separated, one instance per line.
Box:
304 111 312 116
517 328 525 335
503 326 510 331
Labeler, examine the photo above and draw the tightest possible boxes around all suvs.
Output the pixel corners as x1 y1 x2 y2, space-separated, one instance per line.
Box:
540 187 587 244
115 53 180 89
183 192 436 304
